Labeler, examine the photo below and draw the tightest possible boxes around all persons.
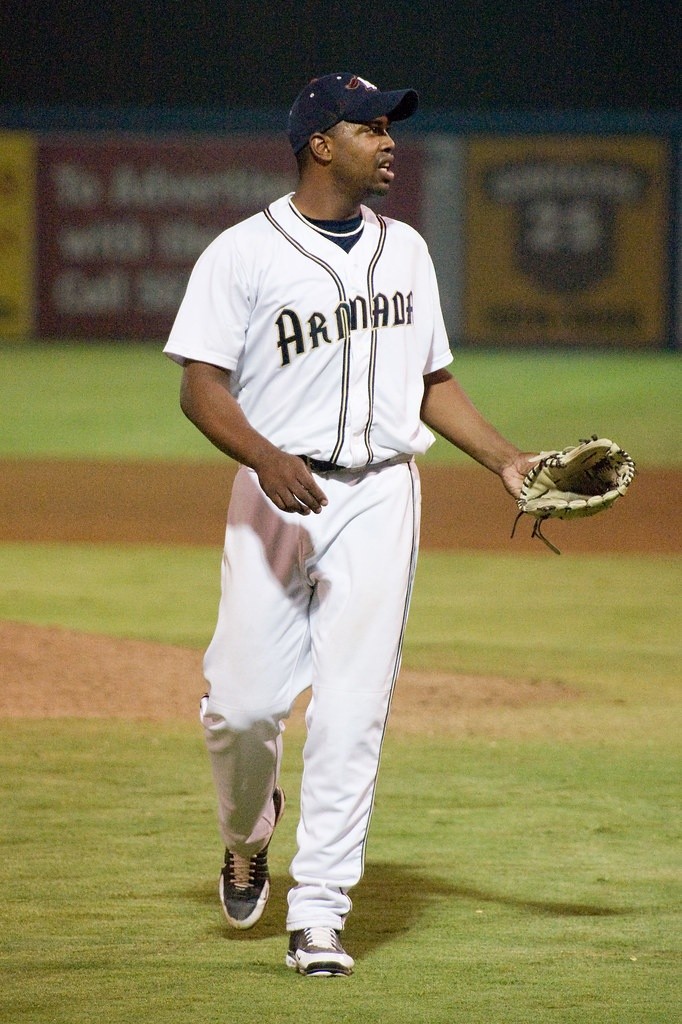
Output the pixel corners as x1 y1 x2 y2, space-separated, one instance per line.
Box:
162 74 542 977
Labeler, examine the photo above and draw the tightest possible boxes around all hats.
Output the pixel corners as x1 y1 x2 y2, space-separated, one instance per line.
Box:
288 71 419 155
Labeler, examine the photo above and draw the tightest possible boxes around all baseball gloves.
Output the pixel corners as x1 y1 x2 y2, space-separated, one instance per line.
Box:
516 439 637 520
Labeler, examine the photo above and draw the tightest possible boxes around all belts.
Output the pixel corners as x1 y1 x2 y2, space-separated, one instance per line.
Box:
300 454 344 472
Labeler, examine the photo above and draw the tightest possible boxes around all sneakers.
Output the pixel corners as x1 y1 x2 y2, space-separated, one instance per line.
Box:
220 788 286 929
286 925 355 978
